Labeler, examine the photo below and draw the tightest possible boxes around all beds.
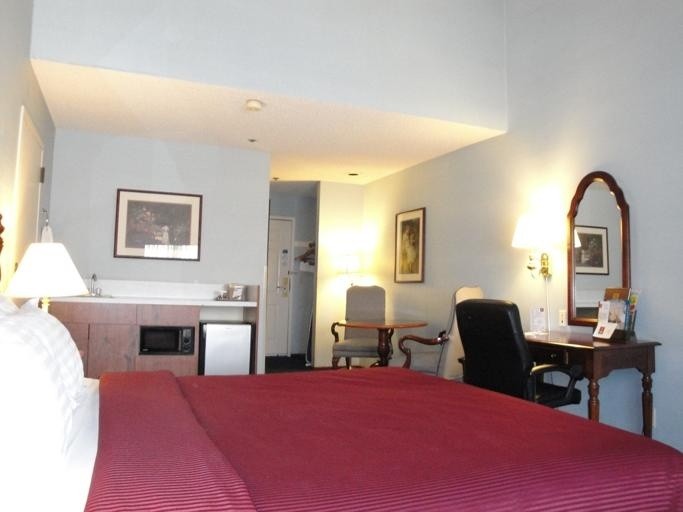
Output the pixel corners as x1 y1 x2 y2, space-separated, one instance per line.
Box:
94 366 682 512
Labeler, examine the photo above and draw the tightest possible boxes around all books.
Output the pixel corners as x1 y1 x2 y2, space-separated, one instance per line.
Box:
593 286 642 339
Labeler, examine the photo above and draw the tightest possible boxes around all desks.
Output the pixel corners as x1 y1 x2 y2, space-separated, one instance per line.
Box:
341 322 429 367
523 330 661 441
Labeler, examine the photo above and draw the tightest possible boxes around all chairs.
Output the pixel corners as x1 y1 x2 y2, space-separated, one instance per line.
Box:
455 300 586 408
331 285 396 370
394 286 482 380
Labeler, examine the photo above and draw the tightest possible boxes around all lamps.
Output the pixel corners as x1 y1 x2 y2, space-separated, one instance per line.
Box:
7 242 89 314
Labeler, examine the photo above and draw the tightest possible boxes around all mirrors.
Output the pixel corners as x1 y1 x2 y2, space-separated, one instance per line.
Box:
563 170 631 326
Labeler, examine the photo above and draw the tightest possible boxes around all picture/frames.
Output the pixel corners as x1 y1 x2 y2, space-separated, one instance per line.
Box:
394 206 425 283
114 188 202 263
573 226 610 275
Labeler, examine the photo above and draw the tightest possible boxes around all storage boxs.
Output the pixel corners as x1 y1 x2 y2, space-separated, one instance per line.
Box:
200 321 256 376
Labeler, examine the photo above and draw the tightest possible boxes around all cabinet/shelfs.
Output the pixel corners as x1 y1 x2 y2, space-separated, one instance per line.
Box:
51 296 200 379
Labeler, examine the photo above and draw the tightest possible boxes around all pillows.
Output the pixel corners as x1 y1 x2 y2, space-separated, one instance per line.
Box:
2 305 87 504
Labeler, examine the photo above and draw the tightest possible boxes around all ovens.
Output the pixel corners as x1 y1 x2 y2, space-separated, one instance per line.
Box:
140 325 195 356
